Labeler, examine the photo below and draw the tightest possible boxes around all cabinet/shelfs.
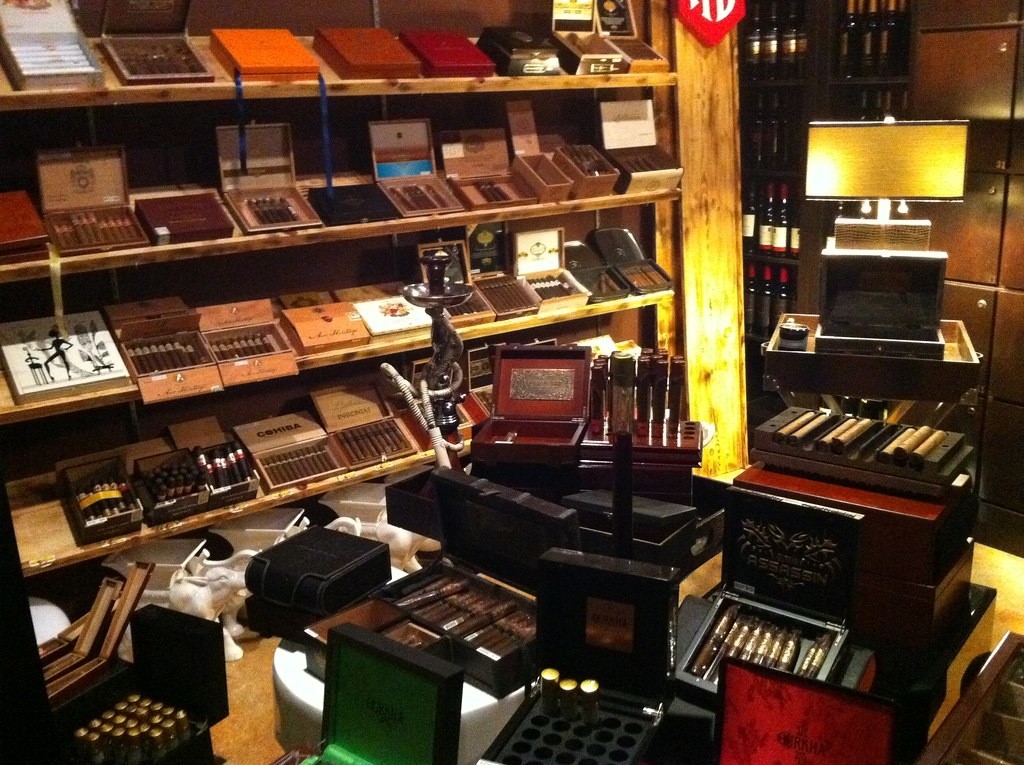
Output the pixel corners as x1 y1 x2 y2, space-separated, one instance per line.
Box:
0 0 684 765
910 0 1024 559
740 0 912 399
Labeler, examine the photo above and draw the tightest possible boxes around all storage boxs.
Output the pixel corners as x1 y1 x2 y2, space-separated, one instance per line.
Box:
0 0 1024 764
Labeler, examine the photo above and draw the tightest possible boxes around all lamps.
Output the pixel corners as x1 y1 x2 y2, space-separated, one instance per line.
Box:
806 113 969 250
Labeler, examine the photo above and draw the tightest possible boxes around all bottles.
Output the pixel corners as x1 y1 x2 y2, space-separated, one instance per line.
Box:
743 179 800 260
857 90 910 120
744 263 798 340
743 0 808 79
836 0 908 78
748 92 800 168
